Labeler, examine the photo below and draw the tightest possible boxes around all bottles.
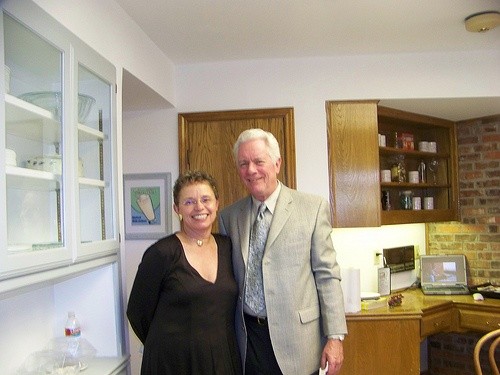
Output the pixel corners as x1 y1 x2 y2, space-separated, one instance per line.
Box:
5 65 13 97
64 311 82 359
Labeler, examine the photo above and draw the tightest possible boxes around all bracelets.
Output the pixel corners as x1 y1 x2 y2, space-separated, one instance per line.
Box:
328 335 345 342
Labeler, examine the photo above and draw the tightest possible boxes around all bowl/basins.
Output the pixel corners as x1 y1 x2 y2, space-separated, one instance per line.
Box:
25 154 83 179
5 148 17 166
18 93 95 124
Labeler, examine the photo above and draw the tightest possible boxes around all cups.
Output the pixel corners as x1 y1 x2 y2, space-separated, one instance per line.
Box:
424 197 434 210
412 197 422 211
380 170 392 183
378 133 386 147
418 141 429 152
429 141 437 153
408 170 420 184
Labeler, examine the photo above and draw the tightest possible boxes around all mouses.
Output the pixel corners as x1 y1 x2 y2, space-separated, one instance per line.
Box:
472 292 484 301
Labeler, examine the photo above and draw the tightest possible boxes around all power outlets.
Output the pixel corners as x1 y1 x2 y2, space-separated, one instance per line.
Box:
373 249 381 265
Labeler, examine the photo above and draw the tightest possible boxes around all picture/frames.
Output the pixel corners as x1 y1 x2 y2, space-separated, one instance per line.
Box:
122 172 173 241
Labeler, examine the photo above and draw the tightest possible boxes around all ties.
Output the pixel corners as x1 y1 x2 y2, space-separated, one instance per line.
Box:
244 204 270 316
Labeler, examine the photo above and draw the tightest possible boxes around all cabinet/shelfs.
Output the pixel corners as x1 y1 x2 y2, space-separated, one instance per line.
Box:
0 0 122 280
0 252 132 375
326 101 461 229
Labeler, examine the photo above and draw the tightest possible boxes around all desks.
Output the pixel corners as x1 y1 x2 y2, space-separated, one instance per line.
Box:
337 285 500 375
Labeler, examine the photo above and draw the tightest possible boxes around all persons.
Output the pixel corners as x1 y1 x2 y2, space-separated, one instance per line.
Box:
218 128 348 375
126 170 247 375
424 262 443 282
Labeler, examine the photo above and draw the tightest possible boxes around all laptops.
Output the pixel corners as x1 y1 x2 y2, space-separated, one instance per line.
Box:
419 255 471 295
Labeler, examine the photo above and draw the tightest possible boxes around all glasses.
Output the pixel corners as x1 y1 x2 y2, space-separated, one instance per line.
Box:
178 196 217 208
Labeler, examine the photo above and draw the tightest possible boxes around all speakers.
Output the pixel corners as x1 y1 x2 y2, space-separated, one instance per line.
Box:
378 267 390 296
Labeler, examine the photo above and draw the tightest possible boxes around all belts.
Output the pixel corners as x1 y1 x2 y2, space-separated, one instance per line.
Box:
243 313 267 326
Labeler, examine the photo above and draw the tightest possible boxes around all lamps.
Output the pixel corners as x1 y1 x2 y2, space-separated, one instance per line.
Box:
465 12 499 32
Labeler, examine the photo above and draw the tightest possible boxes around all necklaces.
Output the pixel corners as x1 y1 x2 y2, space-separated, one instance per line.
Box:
191 238 208 247
